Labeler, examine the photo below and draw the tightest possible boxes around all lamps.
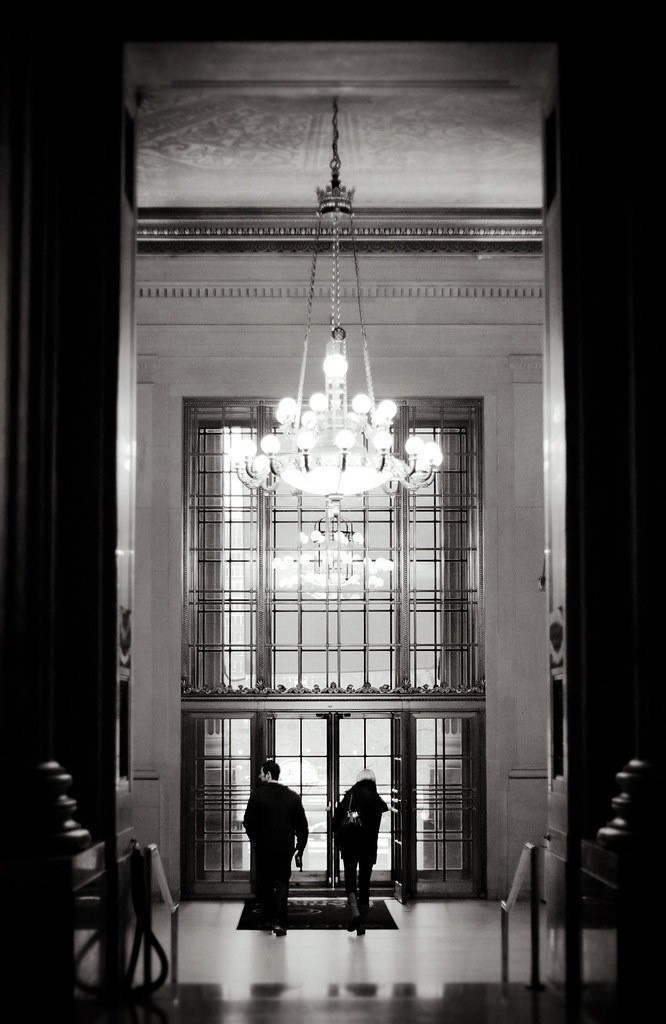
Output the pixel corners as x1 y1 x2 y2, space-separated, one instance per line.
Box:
232 94 444 593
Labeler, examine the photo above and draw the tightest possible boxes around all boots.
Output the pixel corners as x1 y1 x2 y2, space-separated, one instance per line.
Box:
358 896 369 936
347 892 360 932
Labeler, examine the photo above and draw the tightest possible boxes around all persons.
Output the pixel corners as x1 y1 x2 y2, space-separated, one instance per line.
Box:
244 760 309 937
334 769 388 936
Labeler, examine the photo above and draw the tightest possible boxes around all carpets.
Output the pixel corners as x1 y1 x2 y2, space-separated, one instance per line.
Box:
236 896 400 930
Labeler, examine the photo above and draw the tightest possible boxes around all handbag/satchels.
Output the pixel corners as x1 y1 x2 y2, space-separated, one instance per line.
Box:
339 789 363 840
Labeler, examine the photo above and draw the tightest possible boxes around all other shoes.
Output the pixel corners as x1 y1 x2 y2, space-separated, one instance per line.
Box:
274 925 287 937
